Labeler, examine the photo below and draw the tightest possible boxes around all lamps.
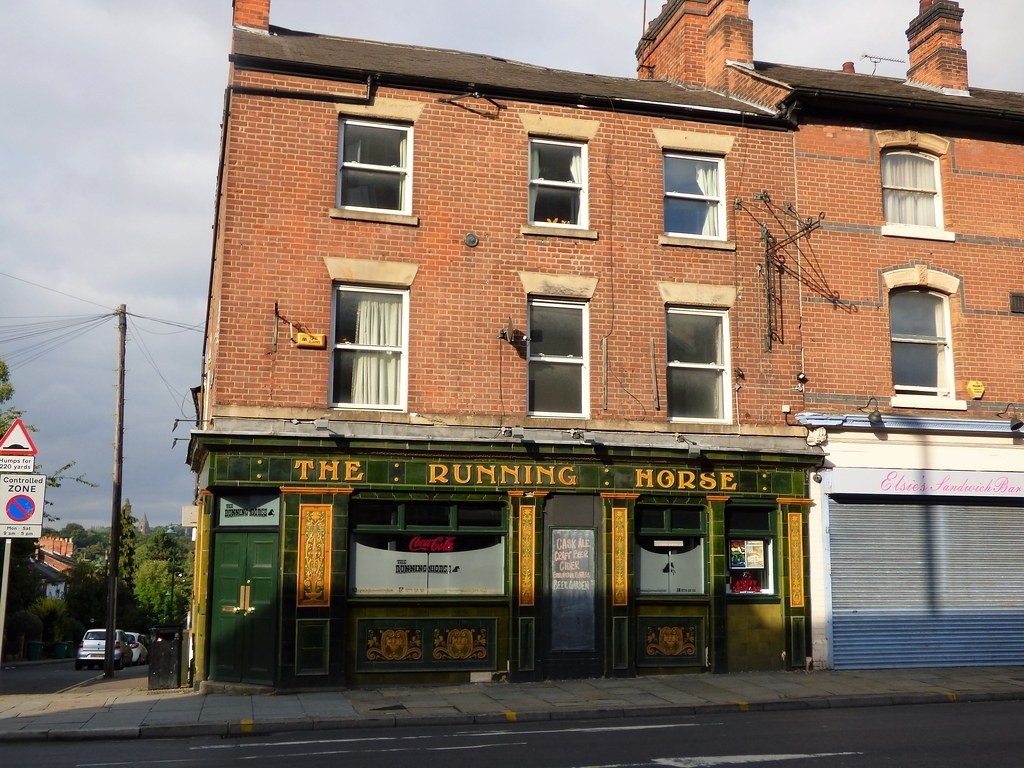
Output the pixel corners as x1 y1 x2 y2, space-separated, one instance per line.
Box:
673 432 701 458
313 419 330 430
995 403 1024 430
572 429 597 443
501 427 526 439
858 396 884 424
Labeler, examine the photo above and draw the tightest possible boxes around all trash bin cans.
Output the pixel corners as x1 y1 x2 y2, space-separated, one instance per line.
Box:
148 626 180 690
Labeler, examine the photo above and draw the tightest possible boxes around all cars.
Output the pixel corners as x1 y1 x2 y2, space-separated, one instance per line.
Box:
75 628 135 670
123 631 149 666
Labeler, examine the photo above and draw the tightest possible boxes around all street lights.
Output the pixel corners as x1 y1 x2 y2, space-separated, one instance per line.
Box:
163 524 176 620
